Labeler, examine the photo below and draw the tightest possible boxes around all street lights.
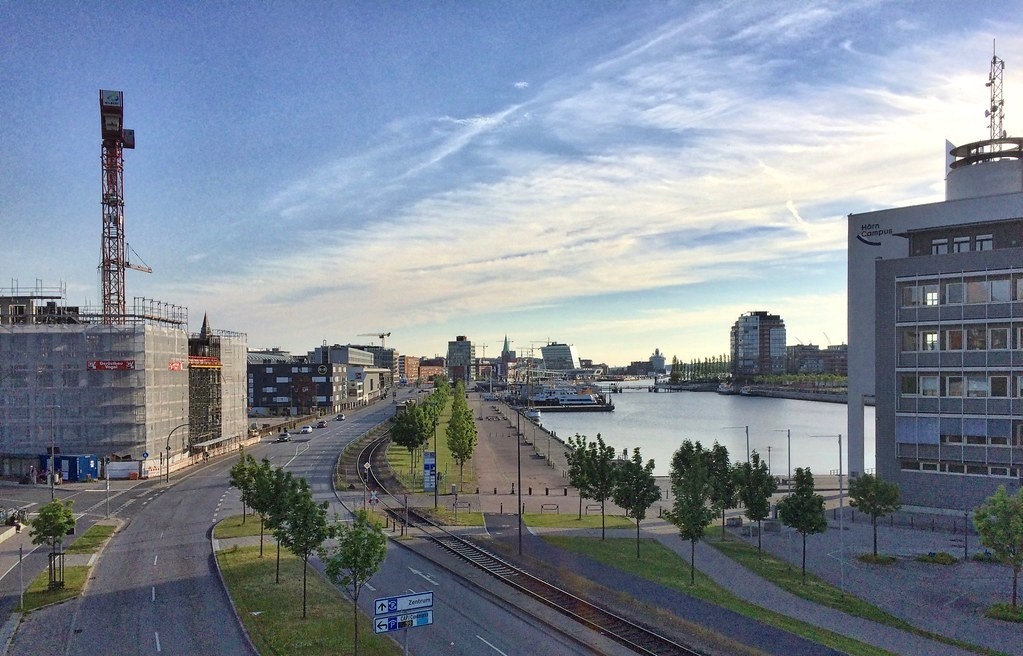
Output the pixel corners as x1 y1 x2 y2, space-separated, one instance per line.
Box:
290 385 294 430
771 428 791 495
47 405 60 500
723 426 762 551
811 433 844 588
315 384 319 420
510 407 525 552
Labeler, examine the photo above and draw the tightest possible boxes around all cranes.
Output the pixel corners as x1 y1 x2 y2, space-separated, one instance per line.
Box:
496 337 557 358
355 332 391 348
476 342 488 358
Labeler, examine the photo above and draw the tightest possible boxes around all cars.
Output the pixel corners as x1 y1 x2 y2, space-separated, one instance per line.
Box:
277 433 292 443
316 420 328 428
337 414 346 421
301 425 313 434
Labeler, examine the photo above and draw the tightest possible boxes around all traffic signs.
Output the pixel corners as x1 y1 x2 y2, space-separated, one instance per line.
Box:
374 591 434 615
373 610 434 634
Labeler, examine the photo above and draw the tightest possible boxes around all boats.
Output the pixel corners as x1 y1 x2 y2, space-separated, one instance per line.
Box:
513 385 615 412
738 379 753 396
716 381 736 394
